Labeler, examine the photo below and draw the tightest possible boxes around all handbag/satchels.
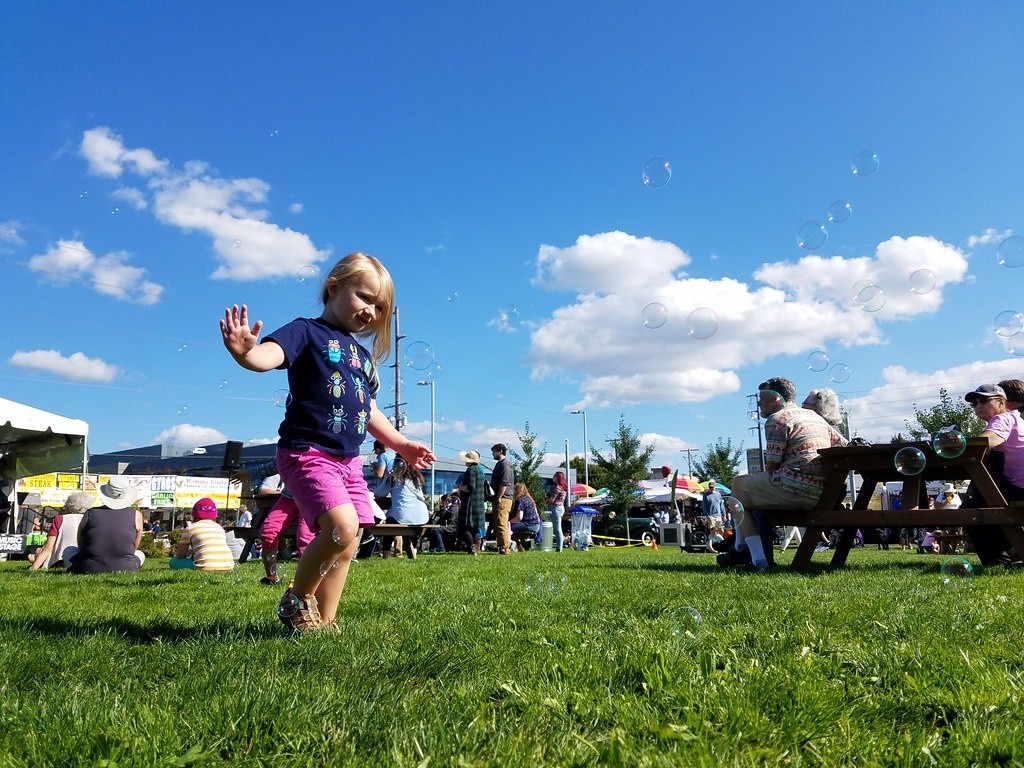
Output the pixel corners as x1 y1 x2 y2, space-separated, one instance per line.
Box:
483 479 496 501
716 547 752 567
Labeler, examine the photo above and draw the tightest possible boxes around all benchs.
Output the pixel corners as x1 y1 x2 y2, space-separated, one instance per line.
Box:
222 525 298 539
366 523 441 536
511 530 536 541
751 507 814 568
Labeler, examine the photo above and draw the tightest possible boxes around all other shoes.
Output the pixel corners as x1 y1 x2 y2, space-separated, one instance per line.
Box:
276 547 290 560
260 575 277 585
28 553 36 563
737 561 772 576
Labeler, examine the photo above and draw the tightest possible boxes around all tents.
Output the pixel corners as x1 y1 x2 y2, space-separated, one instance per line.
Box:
0 397 88 490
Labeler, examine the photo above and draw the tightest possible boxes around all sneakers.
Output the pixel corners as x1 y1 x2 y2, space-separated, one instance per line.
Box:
323 618 342 634
277 579 323 637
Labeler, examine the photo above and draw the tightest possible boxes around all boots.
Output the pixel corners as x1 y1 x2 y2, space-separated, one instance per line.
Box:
467 539 474 556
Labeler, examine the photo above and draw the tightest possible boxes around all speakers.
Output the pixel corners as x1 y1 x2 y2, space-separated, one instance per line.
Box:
224 441 243 470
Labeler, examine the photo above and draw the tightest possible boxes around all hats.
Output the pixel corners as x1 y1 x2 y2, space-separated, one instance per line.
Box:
237 504 248 511
459 449 481 465
94 474 138 510
708 482 715 488
191 498 218 519
964 384 1007 403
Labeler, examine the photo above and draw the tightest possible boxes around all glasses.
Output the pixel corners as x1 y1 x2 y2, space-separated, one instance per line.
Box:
801 401 816 408
969 397 1007 409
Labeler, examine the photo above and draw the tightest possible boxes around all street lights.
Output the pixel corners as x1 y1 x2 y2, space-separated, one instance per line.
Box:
571 409 590 497
417 380 435 517
681 448 699 480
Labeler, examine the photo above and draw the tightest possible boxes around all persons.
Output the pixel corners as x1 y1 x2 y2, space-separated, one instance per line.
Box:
890 483 963 555
63 477 146 572
550 471 568 552
780 526 801 553
965 380 1024 568
429 443 540 553
143 461 315 585
357 441 428 560
712 377 848 572
220 252 437 632
28 493 94 571
651 482 733 553
822 503 863 548
0 458 42 561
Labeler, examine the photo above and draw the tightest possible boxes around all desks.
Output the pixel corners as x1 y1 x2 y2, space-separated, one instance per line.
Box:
237 494 297 563
789 437 1024 575
485 512 526 552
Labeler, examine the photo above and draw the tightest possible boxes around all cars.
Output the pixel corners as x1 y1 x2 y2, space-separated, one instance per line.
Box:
591 504 659 546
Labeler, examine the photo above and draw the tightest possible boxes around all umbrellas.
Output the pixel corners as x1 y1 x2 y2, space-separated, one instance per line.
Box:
593 488 613 496
701 481 731 495
663 477 704 512
646 486 703 503
570 483 596 498
